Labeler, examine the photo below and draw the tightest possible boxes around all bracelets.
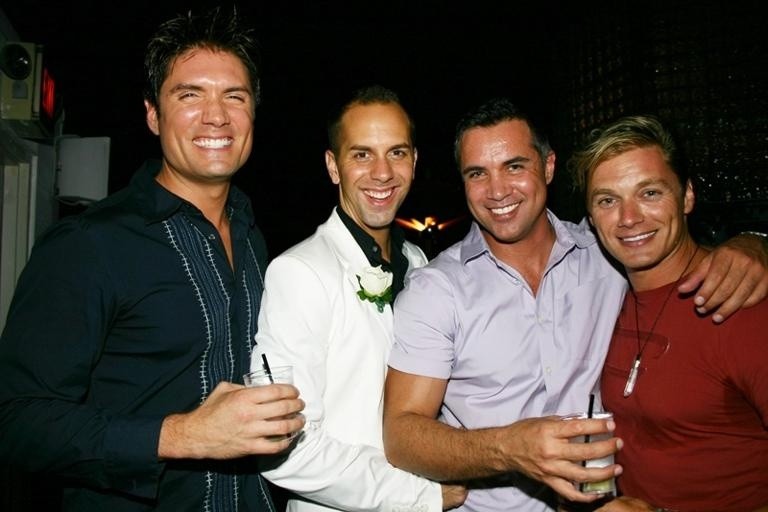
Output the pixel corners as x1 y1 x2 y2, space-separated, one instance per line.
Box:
739 228 768 241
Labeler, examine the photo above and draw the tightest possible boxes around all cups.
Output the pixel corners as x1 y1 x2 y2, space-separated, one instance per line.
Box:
561 411 615 496
243 365 300 443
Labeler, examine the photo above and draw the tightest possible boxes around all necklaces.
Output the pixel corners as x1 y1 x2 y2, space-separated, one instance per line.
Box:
621 243 700 401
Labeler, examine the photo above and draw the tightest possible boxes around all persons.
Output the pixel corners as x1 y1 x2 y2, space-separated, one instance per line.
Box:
248 86 471 511
1 4 306 512
381 96 768 512
556 112 767 512
